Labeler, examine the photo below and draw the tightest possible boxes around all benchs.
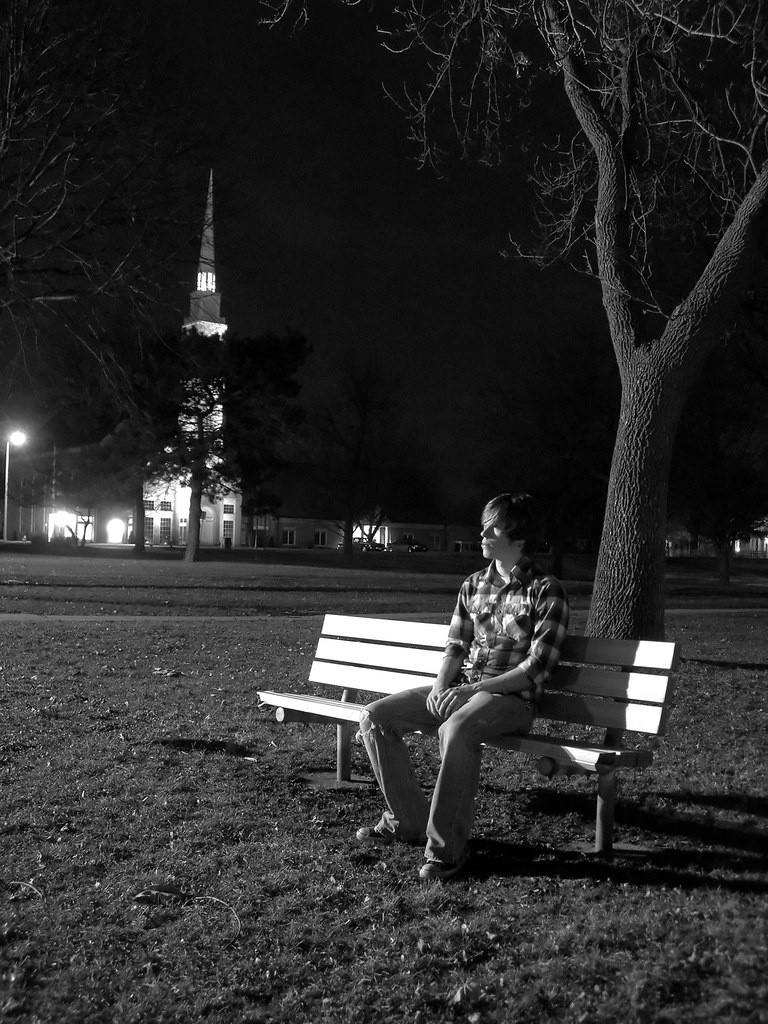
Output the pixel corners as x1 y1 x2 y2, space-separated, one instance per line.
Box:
256 613 680 856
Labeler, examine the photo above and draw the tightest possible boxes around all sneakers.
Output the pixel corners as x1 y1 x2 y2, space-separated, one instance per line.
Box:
419 850 468 879
355 826 388 841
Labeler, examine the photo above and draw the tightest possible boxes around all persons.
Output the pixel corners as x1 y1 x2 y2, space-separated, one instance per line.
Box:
356 492 570 877
